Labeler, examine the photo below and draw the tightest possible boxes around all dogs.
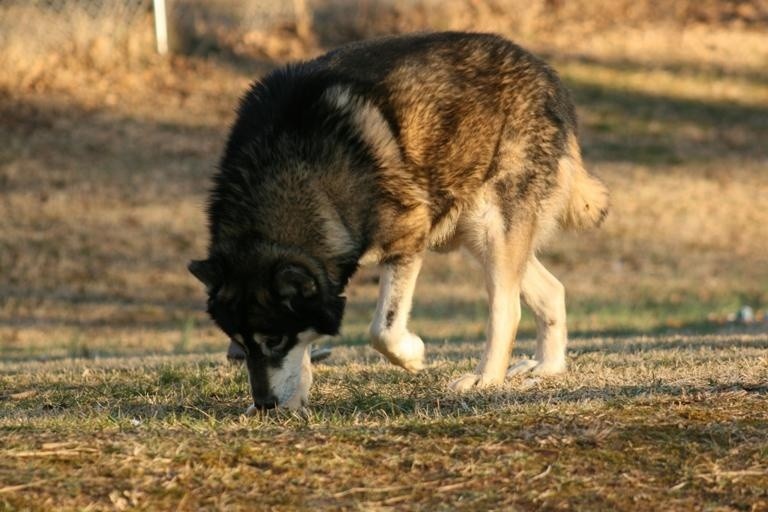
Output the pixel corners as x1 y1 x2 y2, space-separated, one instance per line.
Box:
186 31 610 415
